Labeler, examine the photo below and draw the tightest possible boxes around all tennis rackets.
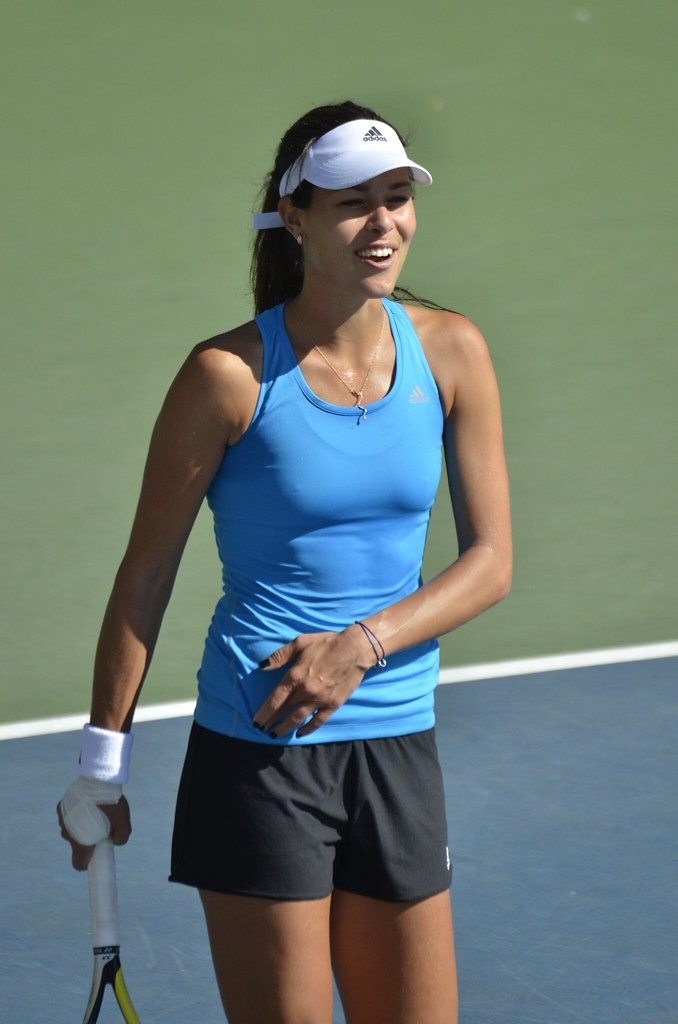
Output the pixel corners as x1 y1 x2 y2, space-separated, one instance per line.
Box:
81 837 144 1024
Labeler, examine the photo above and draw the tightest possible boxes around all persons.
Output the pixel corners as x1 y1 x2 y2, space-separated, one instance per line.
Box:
58 101 512 1024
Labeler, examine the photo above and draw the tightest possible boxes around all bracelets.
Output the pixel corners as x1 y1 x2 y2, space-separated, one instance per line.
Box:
356 620 387 669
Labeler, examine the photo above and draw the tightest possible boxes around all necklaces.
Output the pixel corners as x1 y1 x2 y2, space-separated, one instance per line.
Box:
292 298 385 420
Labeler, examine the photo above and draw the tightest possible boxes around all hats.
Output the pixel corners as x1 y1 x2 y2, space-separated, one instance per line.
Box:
279 119 434 198
